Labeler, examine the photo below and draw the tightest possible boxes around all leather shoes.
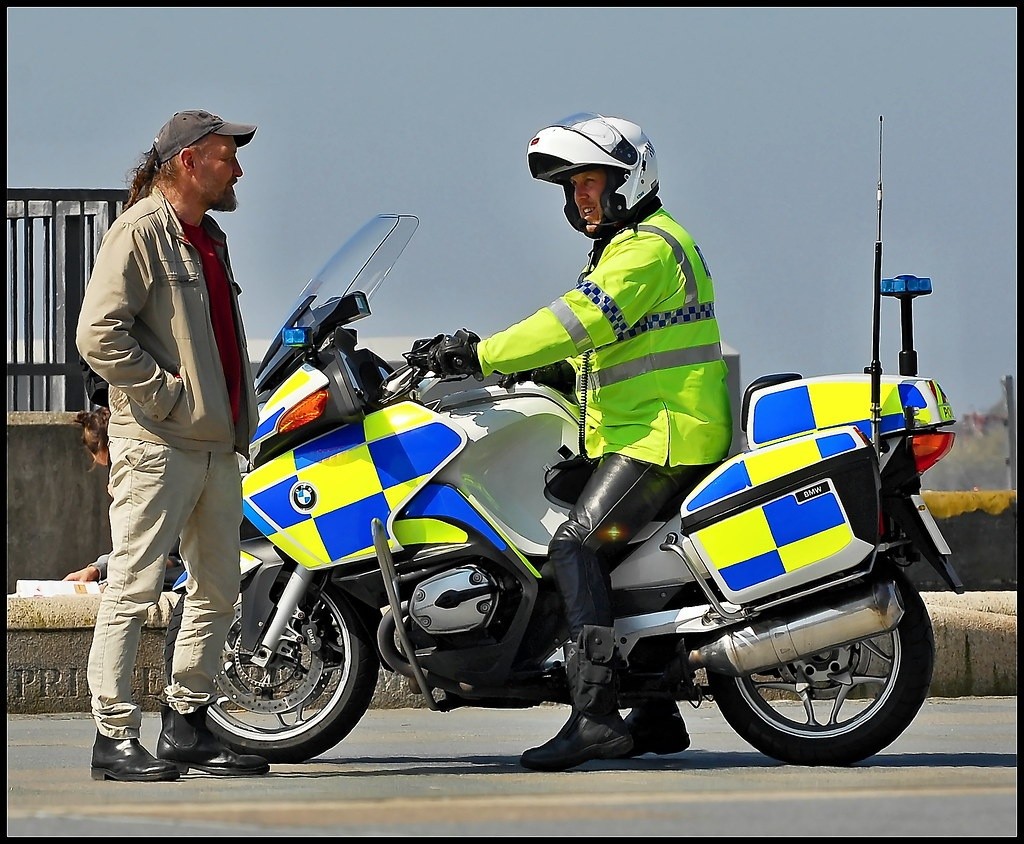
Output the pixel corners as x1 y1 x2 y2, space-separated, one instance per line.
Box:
91 727 180 782
157 704 270 777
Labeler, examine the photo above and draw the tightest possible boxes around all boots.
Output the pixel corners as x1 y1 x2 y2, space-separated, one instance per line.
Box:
520 624 690 772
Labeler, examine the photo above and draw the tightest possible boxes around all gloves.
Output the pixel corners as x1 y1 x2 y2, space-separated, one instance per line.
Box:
428 328 481 379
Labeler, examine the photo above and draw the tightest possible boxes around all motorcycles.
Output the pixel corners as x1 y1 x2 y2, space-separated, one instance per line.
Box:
161 114 964 769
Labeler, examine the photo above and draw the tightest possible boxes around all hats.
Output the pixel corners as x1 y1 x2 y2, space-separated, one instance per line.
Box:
153 109 258 171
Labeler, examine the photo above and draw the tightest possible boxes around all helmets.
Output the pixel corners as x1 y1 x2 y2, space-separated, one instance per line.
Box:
526 111 659 240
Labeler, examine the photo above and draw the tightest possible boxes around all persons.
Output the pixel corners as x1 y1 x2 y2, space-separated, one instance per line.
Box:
76 110 271 786
409 110 729 773
65 409 188 589
